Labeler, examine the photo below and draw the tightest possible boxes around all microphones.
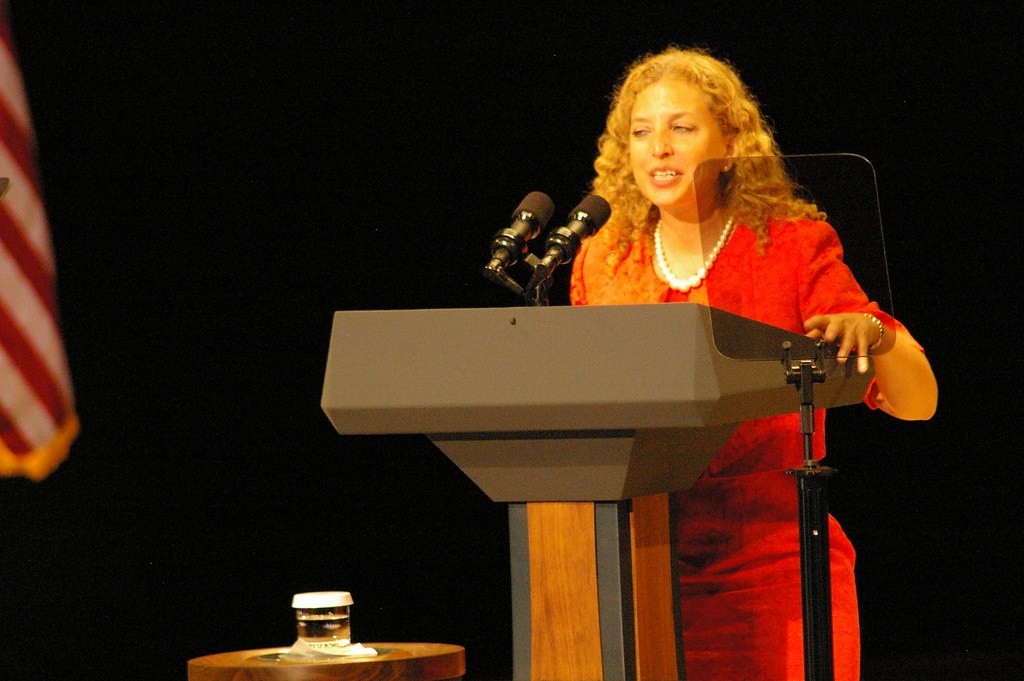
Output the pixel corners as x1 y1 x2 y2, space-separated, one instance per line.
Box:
526 194 612 293
483 190 555 280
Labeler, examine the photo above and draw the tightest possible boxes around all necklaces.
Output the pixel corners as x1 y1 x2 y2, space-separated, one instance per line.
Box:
654 215 736 294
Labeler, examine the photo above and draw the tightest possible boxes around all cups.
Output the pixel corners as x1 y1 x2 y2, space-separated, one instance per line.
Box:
291 591 354 648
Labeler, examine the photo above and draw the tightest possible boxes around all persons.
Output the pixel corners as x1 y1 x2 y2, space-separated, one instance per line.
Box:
569 50 939 680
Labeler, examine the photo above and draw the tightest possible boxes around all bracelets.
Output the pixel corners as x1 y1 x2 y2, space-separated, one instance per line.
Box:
863 310 888 351
